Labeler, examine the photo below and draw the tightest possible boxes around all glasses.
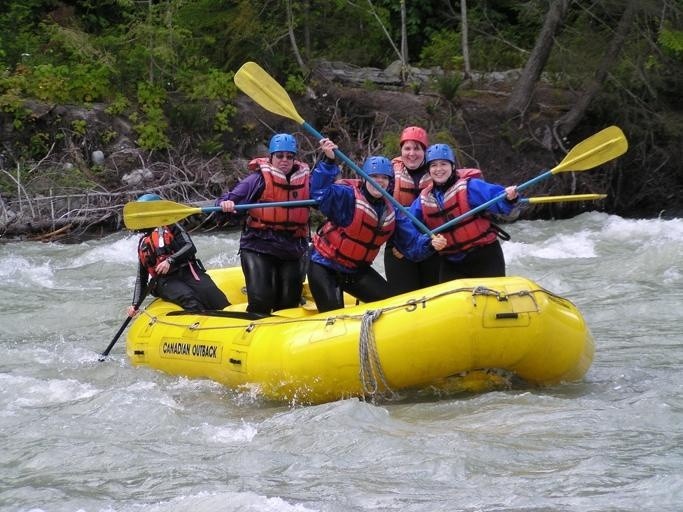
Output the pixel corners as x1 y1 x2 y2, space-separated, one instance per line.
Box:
276 153 295 160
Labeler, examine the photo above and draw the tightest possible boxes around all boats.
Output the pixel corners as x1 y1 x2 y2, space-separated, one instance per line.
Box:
125 266 596 405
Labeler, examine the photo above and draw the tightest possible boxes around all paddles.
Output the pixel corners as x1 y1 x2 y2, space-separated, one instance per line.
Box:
122 199 317 231
234 62 437 240
519 194 608 203
422 126 629 237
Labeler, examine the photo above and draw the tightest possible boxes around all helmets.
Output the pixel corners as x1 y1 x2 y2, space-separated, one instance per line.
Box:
363 156 394 178
138 194 162 202
400 126 429 148
269 134 297 154
426 144 455 163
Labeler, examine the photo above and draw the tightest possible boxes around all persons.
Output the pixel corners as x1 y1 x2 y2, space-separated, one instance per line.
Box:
214 133 311 317
126 194 232 317
384 127 432 296
392 144 520 284
307 137 447 313
